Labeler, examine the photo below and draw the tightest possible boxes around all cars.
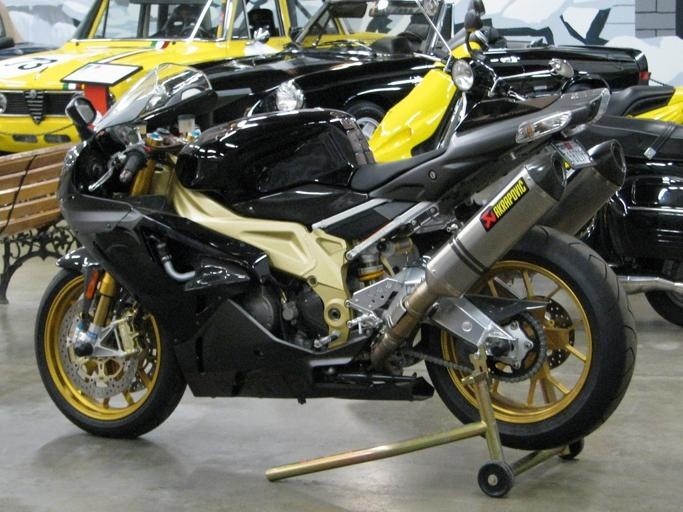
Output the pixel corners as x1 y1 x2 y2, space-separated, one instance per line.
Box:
1 0 394 158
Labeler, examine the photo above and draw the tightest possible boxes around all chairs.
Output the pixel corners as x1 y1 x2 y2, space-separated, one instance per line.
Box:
156 4 275 42
395 20 503 47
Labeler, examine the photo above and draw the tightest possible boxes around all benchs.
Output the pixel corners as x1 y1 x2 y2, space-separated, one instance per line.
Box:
0 141 81 303
